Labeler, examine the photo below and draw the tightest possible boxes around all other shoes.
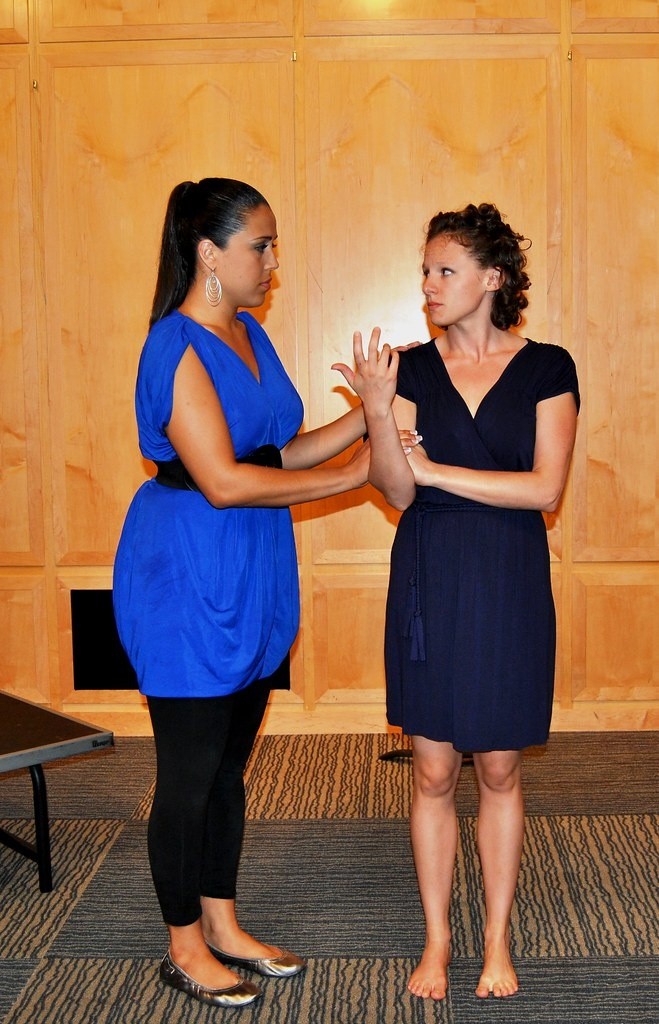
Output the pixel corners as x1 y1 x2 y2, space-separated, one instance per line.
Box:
160 949 262 1007
203 939 305 977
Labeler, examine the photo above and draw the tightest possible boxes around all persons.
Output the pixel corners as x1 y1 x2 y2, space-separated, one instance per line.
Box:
330 203 581 998
111 178 422 1013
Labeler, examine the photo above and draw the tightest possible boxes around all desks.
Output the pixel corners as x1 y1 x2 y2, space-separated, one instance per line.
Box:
0 689 115 895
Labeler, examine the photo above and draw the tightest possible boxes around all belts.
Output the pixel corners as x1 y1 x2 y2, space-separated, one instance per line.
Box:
153 443 283 492
